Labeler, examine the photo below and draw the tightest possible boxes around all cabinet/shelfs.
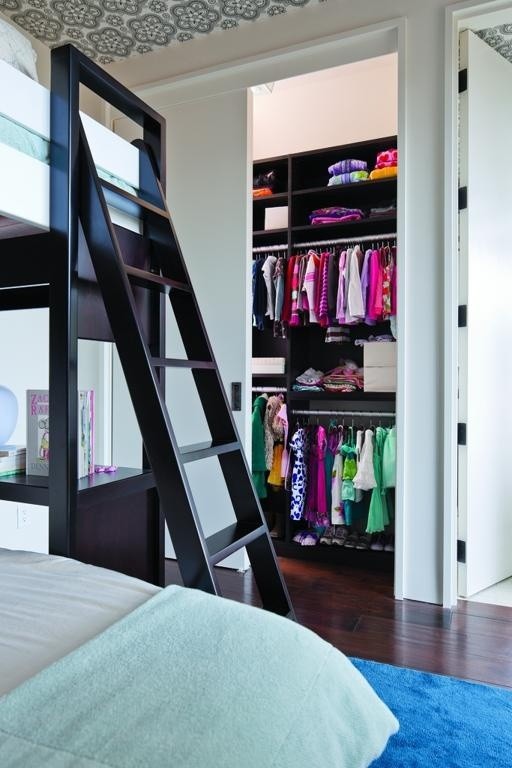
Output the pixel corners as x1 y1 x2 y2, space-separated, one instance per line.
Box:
251 135 396 552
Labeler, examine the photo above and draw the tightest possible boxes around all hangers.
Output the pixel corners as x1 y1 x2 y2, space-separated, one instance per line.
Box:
292 407 394 427
293 233 395 254
252 384 286 397
254 246 287 259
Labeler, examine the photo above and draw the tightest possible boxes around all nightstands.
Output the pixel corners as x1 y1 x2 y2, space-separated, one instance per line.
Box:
0 288 166 506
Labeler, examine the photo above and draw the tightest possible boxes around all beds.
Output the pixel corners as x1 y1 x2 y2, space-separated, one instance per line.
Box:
0 545 401 768
1 44 165 240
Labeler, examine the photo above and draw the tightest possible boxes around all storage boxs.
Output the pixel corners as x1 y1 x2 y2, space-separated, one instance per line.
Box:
365 341 397 392
252 358 286 374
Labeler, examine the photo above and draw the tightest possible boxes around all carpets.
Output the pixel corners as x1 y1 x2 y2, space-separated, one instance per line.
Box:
342 657 512 767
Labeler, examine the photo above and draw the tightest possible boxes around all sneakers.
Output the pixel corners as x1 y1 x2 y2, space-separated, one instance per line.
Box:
332 527 348 546
320 526 334 546
370 533 384 551
384 534 393 551
344 531 359 548
356 535 369 550
